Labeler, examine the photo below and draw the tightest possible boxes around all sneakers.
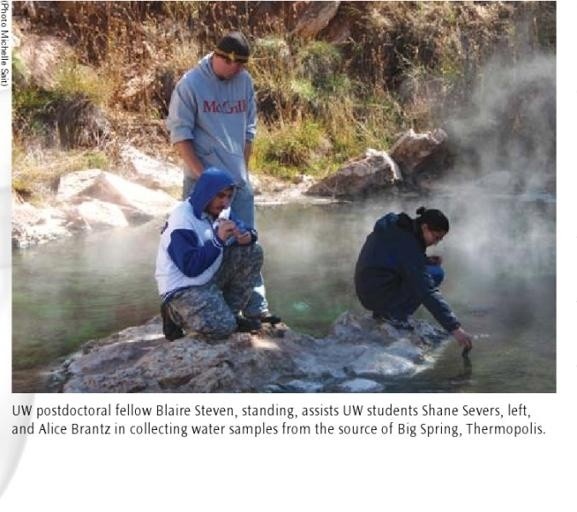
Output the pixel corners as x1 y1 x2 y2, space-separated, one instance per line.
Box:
235 315 260 332
161 304 183 339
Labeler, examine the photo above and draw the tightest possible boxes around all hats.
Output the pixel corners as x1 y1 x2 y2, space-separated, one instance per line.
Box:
216 32 249 62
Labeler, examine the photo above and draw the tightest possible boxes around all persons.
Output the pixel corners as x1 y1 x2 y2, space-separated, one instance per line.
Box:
355 206 473 351
166 30 281 324
156 166 264 340
